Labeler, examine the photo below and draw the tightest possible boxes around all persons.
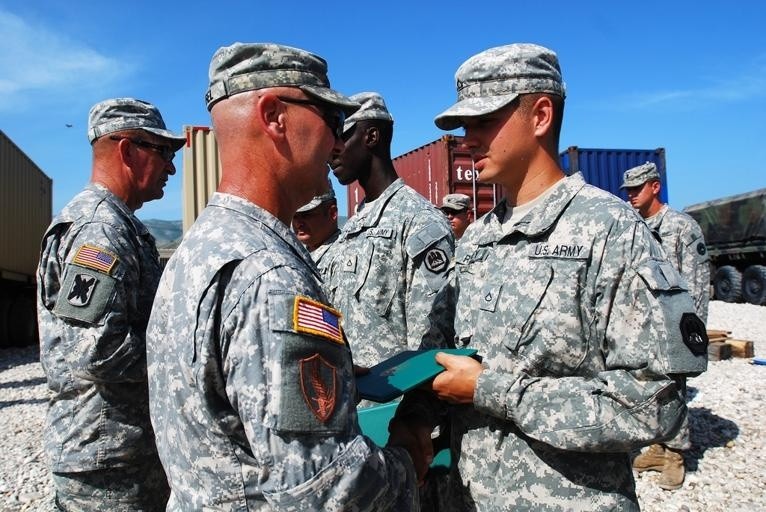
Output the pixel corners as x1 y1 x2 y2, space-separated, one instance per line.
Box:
424 42 707 510
291 178 342 279
27 96 186 512
437 193 471 241
143 43 429 512
618 163 710 490
327 91 454 510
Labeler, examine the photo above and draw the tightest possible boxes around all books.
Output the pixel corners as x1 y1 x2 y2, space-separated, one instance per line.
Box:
350 347 480 405
340 402 454 475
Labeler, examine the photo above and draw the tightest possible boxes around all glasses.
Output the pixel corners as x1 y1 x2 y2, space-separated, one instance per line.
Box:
109 135 176 164
258 94 346 139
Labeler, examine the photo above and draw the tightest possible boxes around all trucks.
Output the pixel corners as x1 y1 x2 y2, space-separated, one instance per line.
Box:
678 187 766 308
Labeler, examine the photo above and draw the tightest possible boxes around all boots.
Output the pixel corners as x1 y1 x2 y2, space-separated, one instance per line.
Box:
658 446 686 490
631 443 666 473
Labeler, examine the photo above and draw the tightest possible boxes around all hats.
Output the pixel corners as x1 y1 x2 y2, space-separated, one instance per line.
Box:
433 42 568 132
295 178 337 214
440 193 471 210
341 91 395 136
618 160 661 191
204 41 362 121
86 96 188 153
